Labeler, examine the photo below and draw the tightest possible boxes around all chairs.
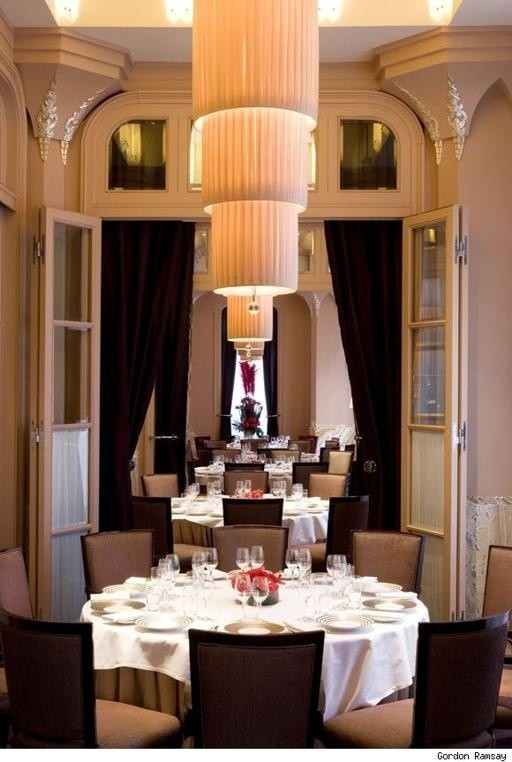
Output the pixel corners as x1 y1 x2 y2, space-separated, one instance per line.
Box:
288 440 312 453
213 524 289 572
482 545 512 664
0 545 34 619
131 496 214 568
0 608 181 749
141 474 178 497
203 440 226 449
292 462 328 489
270 449 300 461
299 436 318 453
320 447 338 462
295 496 369 570
309 473 348 500
224 469 269 495
211 449 241 461
325 441 339 447
182 629 325 749
345 445 355 487
324 608 512 748
329 451 354 473
194 436 211 459
348 529 426 598
491 663 512 730
332 438 339 441
222 498 284 526
80 529 157 600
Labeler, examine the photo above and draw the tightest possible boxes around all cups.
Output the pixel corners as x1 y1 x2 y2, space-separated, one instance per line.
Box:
184 569 200 616
147 566 169 601
249 545 265 570
273 481 280 496
235 480 244 496
158 557 174 582
333 553 347 578
333 577 357 607
206 480 223 511
235 546 249 572
280 480 287 496
245 478 252 494
326 555 334 577
181 482 203 509
285 546 299 589
166 552 182 578
202 546 217 576
189 550 203 578
234 433 291 450
296 548 311 588
291 482 304 500
233 574 251 616
251 575 270 617
212 442 295 477
199 577 215 622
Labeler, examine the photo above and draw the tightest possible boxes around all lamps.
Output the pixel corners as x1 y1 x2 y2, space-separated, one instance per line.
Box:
192 0 318 360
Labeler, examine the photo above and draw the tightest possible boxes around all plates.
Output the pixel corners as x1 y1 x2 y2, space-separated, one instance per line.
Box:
362 582 401 595
172 501 223 519
102 582 153 599
101 609 144 626
135 611 192 631
285 499 330 516
315 611 372 632
359 597 418 614
92 597 145 615
194 493 209 502
223 618 286 636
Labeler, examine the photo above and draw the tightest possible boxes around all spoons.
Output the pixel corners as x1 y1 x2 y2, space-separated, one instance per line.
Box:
281 620 301 633
